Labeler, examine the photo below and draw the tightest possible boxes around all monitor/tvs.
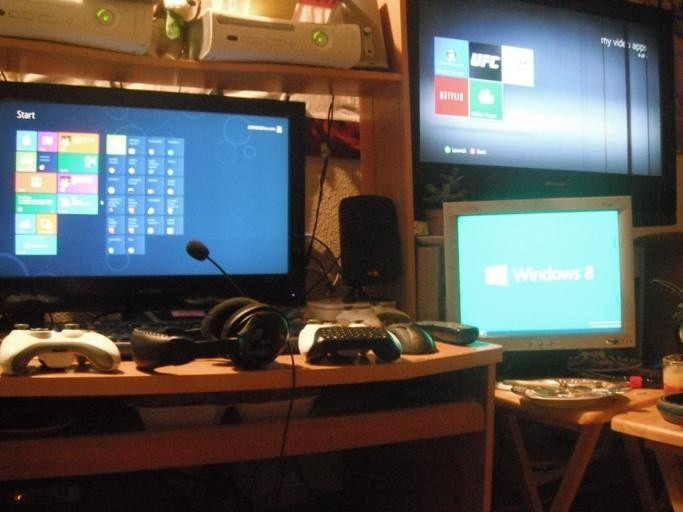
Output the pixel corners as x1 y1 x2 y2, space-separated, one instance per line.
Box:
408 1 677 227
443 195 638 378
0 81 305 313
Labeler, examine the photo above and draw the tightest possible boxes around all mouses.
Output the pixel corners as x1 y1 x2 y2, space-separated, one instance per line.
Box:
387 320 438 354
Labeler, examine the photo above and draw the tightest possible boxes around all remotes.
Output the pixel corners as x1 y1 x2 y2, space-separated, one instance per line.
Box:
417 320 478 346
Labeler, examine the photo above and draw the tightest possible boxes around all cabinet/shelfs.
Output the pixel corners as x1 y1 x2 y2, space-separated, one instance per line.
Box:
0 1 417 324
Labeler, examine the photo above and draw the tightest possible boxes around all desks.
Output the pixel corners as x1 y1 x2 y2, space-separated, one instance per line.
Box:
495 375 683 512
0 339 502 512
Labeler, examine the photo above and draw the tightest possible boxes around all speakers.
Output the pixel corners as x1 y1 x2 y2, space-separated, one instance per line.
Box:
339 193 409 300
326 0 389 70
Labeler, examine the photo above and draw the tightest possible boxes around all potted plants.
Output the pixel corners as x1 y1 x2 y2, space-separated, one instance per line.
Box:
423 168 468 234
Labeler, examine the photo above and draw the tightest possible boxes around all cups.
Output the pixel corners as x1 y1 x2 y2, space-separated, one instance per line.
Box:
662 354 683 396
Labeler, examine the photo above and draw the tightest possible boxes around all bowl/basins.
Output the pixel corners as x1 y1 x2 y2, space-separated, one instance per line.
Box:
656 392 683 426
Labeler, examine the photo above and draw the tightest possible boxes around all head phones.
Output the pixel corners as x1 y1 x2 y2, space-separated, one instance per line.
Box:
130 296 290 370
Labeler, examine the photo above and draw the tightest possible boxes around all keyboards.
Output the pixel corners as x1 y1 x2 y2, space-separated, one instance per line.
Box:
56 317 305 359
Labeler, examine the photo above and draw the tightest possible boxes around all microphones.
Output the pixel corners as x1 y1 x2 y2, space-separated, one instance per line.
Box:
186 240 243 297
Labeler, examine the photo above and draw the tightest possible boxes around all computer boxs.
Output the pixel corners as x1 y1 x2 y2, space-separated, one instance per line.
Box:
0 0 154 56
186 9 363 68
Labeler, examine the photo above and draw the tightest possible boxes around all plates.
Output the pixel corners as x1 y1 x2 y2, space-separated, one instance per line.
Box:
512 378 618 407
